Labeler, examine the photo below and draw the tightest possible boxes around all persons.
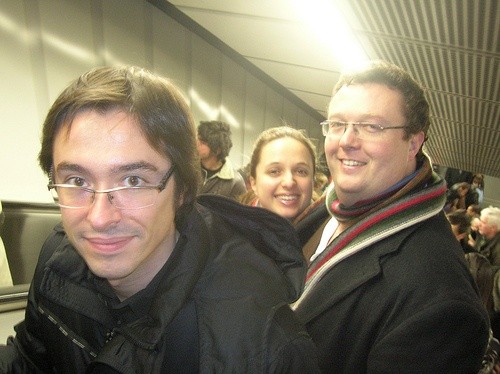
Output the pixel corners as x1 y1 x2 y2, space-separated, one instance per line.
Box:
196 120 247 202
0 65 320 374
238 152 332 198
442 173 500 374
238 127 319 224
287 61 490 374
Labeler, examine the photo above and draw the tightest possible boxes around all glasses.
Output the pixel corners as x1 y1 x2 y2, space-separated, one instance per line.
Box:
320 120 412 136
48 164 177 208
